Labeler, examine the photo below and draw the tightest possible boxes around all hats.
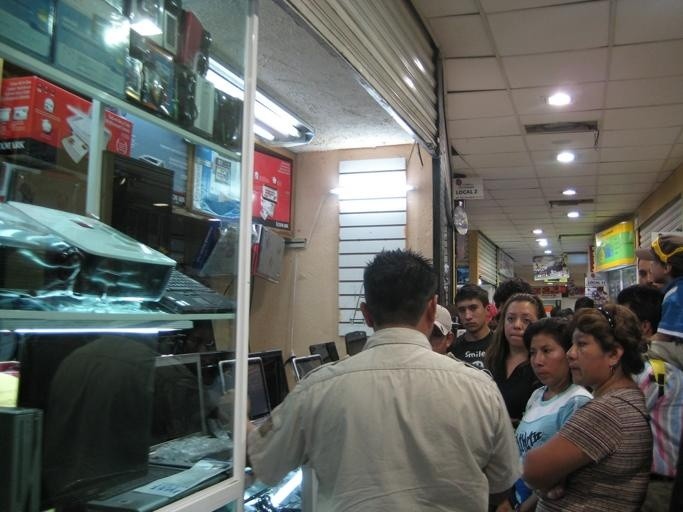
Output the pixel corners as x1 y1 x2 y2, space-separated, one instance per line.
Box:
433 304 452 336
634 234 683 266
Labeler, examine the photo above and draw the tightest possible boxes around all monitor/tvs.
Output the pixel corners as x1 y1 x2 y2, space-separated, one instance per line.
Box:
187 136 293 236
149 320 367 469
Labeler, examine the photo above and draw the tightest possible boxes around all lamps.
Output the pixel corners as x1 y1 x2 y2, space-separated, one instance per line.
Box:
202 55 315 150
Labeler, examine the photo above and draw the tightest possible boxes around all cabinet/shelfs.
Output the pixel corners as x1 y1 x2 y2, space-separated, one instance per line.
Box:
0 0 260 511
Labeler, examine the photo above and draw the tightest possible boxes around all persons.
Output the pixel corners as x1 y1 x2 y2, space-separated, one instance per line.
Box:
429 232 683 510
219 250 522 512
42 335 221 503
